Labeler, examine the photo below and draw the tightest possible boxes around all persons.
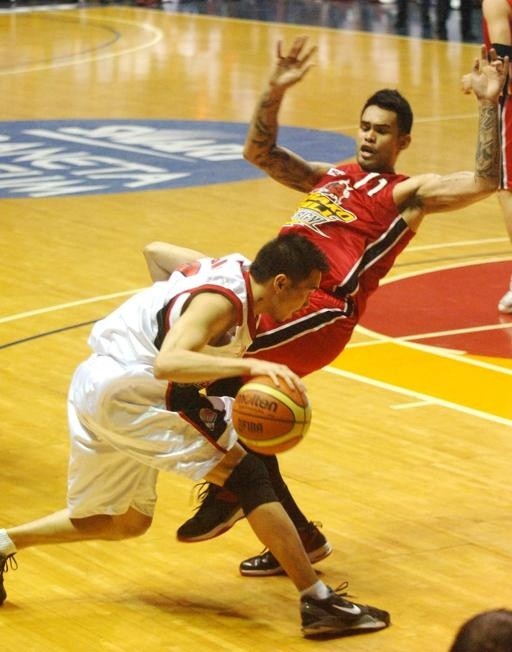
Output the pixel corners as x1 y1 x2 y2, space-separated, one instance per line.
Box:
482 0 512 314
0 234 390 638
178 34 510 578
395 0 478 40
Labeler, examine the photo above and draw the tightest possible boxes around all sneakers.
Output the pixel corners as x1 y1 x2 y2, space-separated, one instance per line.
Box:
300 580 390 637
238 519 333 575
176 480 247 542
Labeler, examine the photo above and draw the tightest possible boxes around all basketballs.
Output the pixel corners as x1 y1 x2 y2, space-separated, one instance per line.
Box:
232 376 312 455
